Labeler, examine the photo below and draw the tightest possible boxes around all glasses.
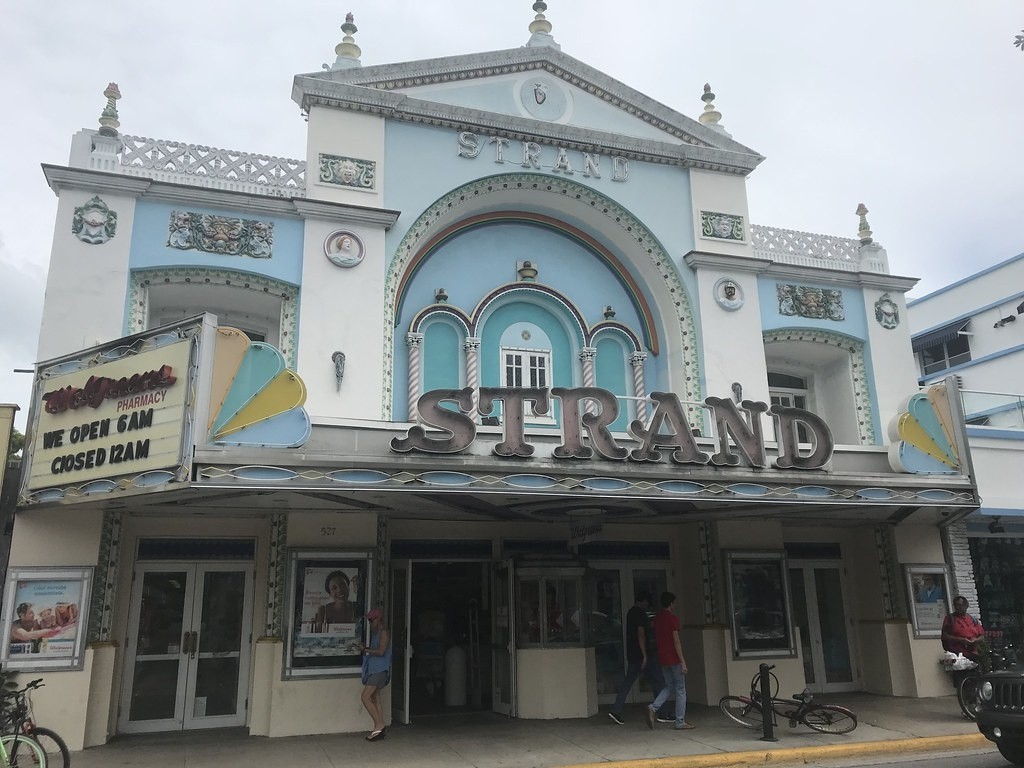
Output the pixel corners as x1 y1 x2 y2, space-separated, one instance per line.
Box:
369 618 376 623
954 603 964 607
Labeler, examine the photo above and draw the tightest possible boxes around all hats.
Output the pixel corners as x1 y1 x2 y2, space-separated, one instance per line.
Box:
637 592 652 606
363 608 384 619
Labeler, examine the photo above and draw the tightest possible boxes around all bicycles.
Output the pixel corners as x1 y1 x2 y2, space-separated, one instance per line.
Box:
719 664 858 734
0 678 71 768
956 643 1021 721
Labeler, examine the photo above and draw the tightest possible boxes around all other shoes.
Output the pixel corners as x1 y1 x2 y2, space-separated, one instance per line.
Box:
365 728 386 742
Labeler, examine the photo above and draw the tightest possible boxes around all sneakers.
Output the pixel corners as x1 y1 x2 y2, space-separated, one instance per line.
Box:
656 712 676 723
646 704 655 730
675 719 694 730
608 711 625 725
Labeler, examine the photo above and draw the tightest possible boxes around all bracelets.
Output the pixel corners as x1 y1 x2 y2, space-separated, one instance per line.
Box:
362 647 366 653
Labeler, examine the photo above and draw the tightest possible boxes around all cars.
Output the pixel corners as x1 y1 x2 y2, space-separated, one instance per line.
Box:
974 655 1024 768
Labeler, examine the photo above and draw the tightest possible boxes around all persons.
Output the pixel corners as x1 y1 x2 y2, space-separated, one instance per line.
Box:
148 583 235 710
316 570 360 633
355 608 393 742
608 591 696 730
532 588 561 641
10 598 78 643
940 596 985 721
916 574 946 603
735 572 780 650
